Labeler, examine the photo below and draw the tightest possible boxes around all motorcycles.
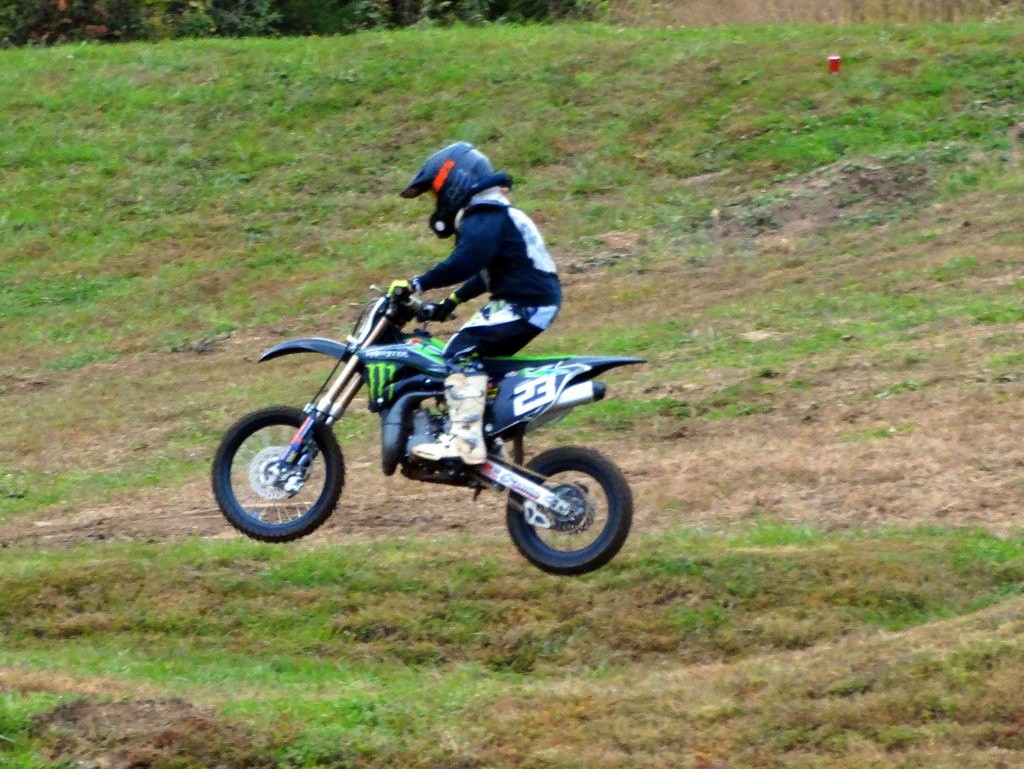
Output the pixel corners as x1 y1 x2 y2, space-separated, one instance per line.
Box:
211 280 648 577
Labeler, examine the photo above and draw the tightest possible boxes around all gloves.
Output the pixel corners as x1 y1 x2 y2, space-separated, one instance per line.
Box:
439 294 458 318
385 279 413 300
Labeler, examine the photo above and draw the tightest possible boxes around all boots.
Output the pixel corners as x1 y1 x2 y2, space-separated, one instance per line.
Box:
413 374 489 468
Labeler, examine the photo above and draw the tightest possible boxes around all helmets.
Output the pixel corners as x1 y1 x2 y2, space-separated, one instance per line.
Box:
398 141 513 238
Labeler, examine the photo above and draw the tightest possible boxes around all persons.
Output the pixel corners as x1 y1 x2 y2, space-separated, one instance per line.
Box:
384 141 562 465
